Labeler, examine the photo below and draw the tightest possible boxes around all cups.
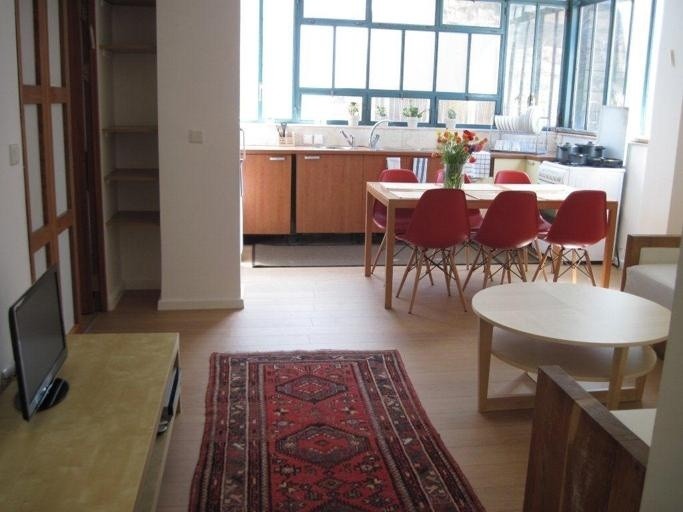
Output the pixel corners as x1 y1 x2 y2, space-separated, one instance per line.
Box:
494 140 520 152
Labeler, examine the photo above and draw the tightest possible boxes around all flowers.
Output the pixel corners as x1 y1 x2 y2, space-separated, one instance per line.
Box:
432 125 487 187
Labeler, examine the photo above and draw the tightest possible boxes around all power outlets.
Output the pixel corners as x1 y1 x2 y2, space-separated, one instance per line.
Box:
8 145 21 165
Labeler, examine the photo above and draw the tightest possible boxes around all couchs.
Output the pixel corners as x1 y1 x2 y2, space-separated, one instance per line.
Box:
523 363 655 512
621 233 682 310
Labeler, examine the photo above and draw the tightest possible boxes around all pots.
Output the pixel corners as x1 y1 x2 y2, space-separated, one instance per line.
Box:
557 141 623 168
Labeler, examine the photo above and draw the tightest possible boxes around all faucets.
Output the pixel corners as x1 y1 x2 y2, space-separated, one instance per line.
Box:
368 119 392 147
340 130 355 147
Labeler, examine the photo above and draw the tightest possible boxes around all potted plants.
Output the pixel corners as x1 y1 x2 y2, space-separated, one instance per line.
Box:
348 102 362 124
373 104 389 126
445 107 457 131
403 103 423 128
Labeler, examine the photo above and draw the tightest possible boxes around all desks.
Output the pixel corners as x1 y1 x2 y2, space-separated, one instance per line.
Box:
0 334 181 512
472 281 673 414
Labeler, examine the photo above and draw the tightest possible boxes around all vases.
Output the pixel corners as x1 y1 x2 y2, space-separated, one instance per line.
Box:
444 162 463 193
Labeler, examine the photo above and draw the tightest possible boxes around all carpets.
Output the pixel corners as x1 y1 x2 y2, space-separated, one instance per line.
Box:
188 349 483 510
252 243 553 267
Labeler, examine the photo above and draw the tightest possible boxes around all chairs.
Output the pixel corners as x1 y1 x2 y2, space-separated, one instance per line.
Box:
371 169 434 284
437 169 493 283
533 191 608 291
461 190 541 291
494 171 555 281
397 189 469 314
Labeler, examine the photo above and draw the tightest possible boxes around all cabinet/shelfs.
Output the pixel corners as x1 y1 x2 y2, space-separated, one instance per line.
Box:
491 153 525 182
241 150 294 236
92 0 243 312
364 152 443 234
296 154 363 234
523 155 556 187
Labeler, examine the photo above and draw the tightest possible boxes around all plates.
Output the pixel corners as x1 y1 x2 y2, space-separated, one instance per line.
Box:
494 105 545 135
501 134 545 154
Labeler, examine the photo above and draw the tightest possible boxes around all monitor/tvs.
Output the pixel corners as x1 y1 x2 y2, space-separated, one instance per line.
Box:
8 259 70 422
